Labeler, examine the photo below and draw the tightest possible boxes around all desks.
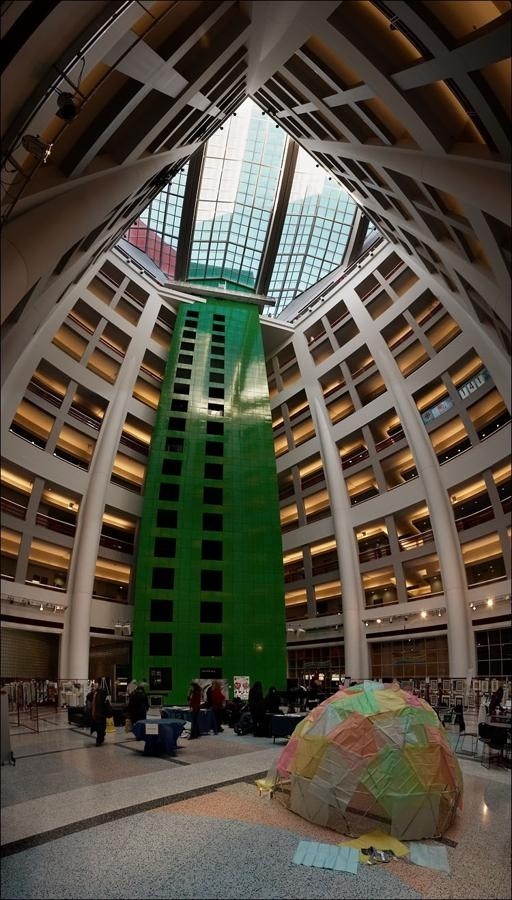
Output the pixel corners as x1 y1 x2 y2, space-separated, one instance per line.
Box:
68 704 123 730
272 712 311 745
138 706 215 757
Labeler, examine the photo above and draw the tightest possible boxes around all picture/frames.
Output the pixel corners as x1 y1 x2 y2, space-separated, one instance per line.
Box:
473 680 498 692
428 679 464 707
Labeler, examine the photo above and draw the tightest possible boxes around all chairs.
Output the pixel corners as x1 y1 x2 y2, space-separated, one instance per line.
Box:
453 704 511 769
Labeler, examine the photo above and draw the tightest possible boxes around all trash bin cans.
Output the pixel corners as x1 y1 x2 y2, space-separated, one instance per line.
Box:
0 690 16 766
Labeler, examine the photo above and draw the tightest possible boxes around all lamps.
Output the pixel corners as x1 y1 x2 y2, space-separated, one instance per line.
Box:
39 603 44 611
437 609 442 617
389 614 408 624
0 54 87 184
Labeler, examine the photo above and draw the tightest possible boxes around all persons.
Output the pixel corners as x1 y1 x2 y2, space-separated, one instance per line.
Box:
125 686 150 742
296 682 307 706
210 679 226 732
139 677 151 695
206 680 216 707
248 678 266 738
126 679 138 695
79 676 114 747
263 685 282 715
488 685 506 713
187 682 204 740
228 695 248 730
